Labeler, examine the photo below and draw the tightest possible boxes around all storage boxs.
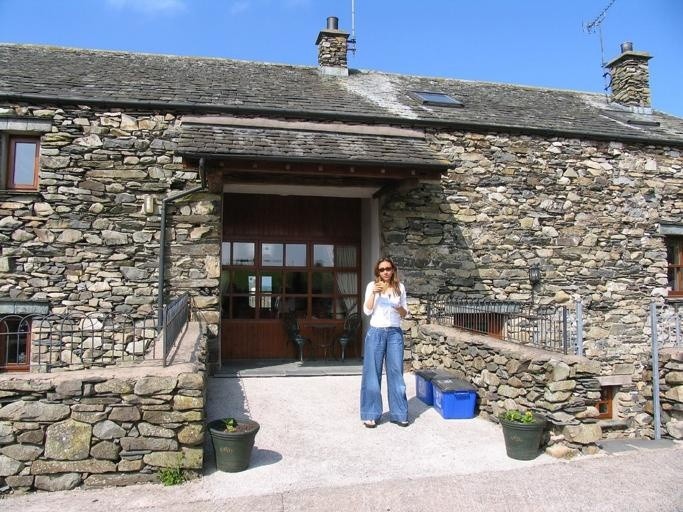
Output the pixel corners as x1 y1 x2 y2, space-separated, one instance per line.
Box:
414 367 479 419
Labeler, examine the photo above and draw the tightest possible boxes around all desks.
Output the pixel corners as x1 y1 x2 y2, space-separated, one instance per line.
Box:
304 322 339 363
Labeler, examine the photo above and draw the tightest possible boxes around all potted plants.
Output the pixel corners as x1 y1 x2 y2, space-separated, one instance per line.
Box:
498 410 548 461
208 418 260 473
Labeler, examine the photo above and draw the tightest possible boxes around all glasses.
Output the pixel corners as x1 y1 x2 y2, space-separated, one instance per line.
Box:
379 268 393 272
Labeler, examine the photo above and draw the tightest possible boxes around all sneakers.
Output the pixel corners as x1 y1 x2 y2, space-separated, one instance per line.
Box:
364 420 376 428
389 420 409 426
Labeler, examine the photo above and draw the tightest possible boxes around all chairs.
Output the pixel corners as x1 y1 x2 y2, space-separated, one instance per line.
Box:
282 313 315 363
330 313 363 362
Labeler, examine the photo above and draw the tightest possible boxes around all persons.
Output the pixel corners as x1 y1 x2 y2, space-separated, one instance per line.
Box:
275 286 295 319
360 258 409 428
